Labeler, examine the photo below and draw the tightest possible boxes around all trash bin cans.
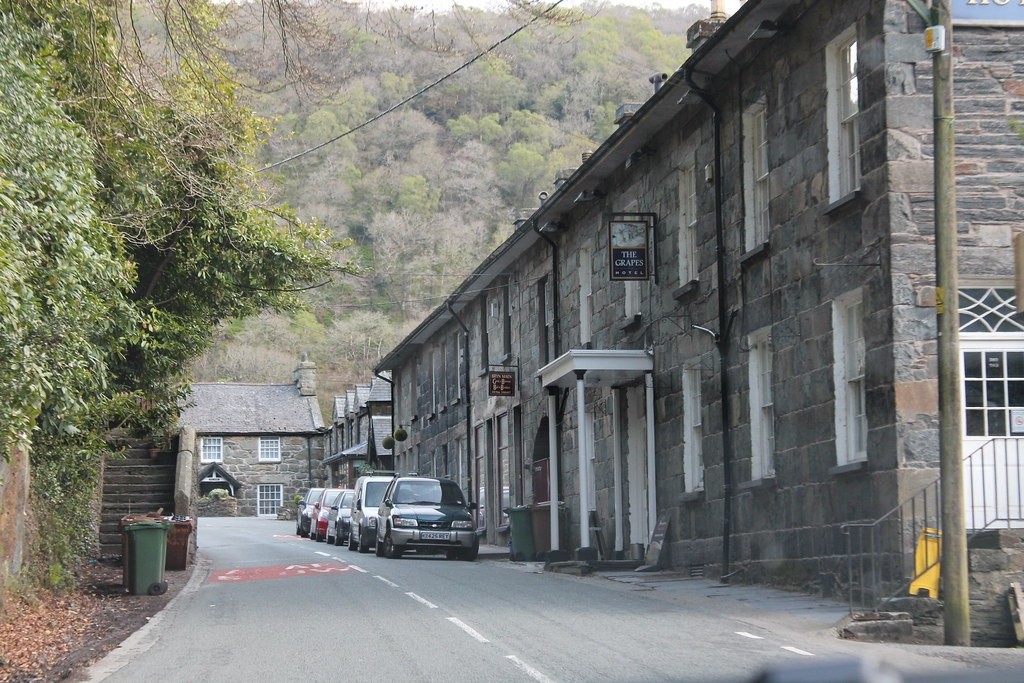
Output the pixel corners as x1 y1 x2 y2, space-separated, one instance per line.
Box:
528 500 570 559
162 518 193 572
503 504 533 562
120 513 171 596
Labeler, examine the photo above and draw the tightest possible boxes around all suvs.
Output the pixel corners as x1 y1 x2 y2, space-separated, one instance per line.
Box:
348 471 401 553
376 473 479 561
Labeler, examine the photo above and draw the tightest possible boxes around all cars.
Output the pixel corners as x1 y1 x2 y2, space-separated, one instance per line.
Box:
296 488 325 538
310 488 343 541
326 491 356 546
